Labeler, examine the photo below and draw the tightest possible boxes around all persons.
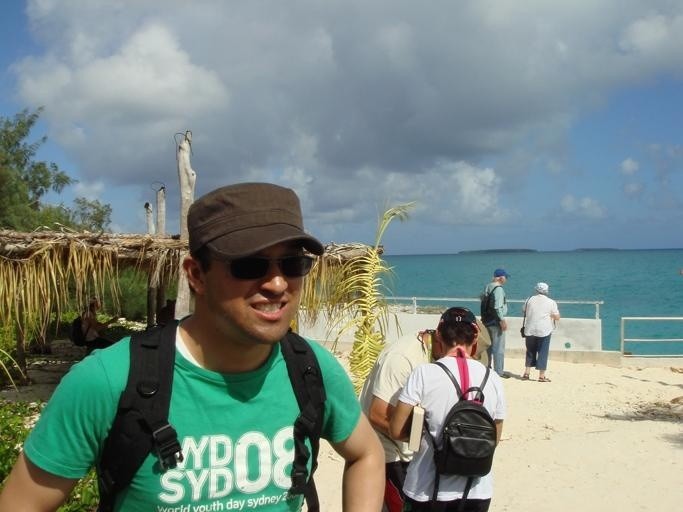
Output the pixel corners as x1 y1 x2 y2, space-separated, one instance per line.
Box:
480 267 512 378
79 296 119 348
356 318 492 512
391 308 506 512
156 300 175 325
519 281 558 382
0 180 386 512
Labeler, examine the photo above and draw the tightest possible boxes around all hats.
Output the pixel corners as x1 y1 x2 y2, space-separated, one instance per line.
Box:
439 307 480 330
494 269 511 277
187 182 323 256
536 282 548 294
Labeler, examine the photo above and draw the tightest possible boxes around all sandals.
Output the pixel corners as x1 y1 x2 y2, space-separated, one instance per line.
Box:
539 377 551 382
524 374 528 378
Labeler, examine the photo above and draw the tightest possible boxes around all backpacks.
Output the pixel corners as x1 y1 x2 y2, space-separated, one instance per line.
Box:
481 285 505 322
422 362 497 476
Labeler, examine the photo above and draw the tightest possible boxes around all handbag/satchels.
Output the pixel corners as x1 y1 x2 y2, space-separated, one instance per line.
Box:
521 327 525 337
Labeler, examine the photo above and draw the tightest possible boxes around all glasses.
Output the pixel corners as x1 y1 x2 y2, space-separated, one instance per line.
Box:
230 255 314 279
